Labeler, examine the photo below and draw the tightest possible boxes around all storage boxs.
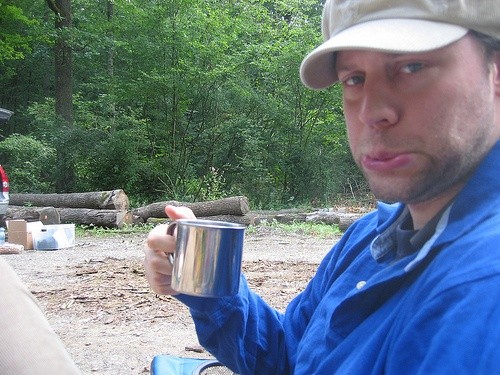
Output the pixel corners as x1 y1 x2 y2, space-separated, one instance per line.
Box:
33 224 75 251
6 219 43 250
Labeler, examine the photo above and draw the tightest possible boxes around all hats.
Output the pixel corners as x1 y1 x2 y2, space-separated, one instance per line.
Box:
299 0 500 92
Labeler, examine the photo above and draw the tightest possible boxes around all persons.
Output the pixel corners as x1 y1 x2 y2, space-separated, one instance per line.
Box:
142 1 500 375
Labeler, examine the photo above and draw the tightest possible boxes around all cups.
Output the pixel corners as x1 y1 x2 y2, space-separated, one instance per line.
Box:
165 219 246 298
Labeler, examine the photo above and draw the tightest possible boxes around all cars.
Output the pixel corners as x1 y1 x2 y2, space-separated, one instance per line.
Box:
0 108 15 226
